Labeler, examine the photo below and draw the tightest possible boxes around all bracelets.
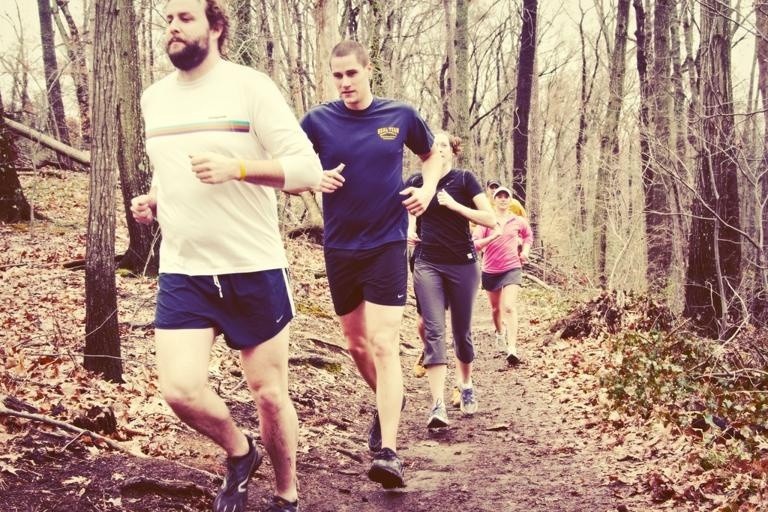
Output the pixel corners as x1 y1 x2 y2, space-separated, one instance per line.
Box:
235 157 245 182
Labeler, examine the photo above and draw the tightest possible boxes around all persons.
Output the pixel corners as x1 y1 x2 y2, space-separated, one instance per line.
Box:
403 130 498 426
487 179 527 221
471 187 533 362
129 0 323 511
293 39 442 489
411 289 462 407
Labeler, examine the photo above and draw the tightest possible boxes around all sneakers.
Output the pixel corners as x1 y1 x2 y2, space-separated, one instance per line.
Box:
487 179 501 190
262 494 298 512
368 395 407 451
495 326 507 353
427 399 448 428
458 383 477 415
494 187 511 200
506 354 519 366
212 435 261 512
368 448 406 488
